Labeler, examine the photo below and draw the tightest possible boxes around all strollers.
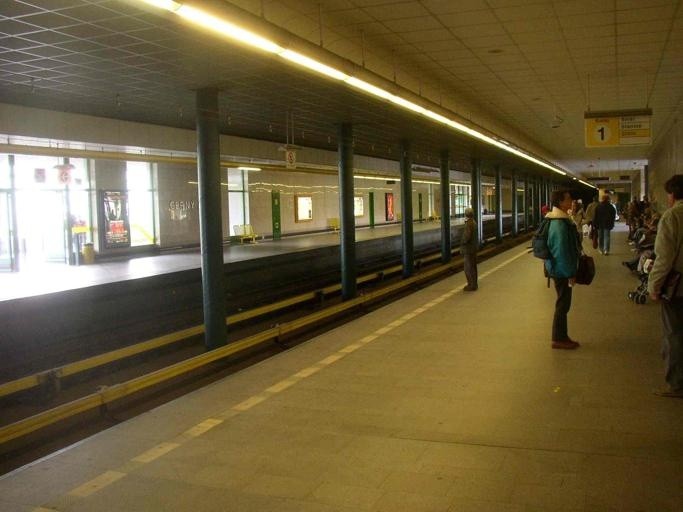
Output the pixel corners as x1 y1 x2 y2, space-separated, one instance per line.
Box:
627 271 669 308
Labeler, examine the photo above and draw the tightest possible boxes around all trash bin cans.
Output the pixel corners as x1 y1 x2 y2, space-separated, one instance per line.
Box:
81 243 95 264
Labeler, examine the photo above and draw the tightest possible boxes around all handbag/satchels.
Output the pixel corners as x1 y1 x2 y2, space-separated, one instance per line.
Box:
589 225 598 249
575 255 596 285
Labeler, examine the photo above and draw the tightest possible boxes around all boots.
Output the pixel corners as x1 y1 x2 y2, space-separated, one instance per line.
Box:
463 269 478 291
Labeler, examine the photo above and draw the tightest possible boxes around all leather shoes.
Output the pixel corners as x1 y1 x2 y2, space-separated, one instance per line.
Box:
551 340 577 349
654 384 683 397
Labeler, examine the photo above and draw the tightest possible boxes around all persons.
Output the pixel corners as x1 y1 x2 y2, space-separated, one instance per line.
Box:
459 208 478 291
531 174 683 399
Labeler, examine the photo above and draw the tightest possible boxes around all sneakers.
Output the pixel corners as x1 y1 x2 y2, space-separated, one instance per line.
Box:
604 249 609 255
598 248 603 255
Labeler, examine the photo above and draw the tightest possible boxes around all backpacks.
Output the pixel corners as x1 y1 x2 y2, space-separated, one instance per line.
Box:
527 217 550 259
